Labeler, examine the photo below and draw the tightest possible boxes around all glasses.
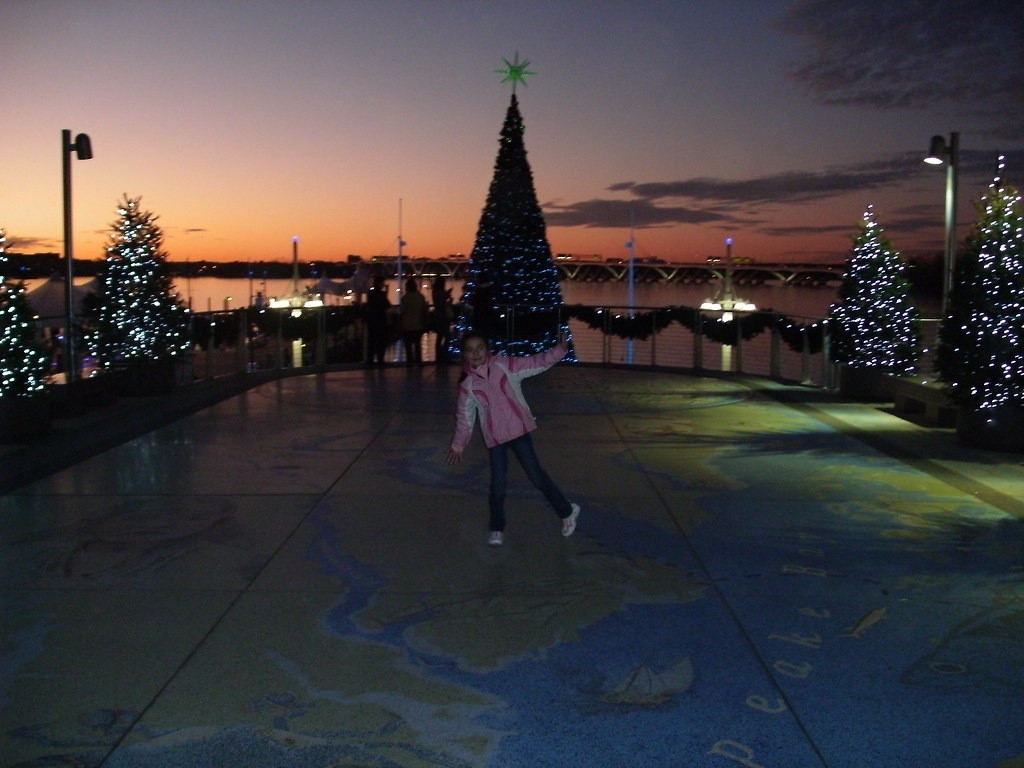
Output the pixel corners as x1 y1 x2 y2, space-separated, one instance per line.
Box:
465 346 485 354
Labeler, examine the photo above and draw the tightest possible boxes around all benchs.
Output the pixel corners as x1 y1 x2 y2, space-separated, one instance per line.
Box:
881 373 967 424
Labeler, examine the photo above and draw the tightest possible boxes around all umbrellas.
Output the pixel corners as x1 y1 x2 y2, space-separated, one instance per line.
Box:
344 269 372 301
20 274 100 341
303 278 345 302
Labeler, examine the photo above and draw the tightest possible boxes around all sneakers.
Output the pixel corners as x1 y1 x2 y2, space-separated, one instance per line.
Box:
488 530 504 546
562 503 580 537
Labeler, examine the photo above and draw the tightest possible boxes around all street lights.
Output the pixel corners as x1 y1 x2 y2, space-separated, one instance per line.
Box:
922 128 959 317
61 127 94 393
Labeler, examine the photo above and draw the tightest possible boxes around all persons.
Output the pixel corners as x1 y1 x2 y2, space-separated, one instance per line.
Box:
253 292 264 309
445 331 580 546
400 280 428 369
365 275 393 373
431 277 454 370
